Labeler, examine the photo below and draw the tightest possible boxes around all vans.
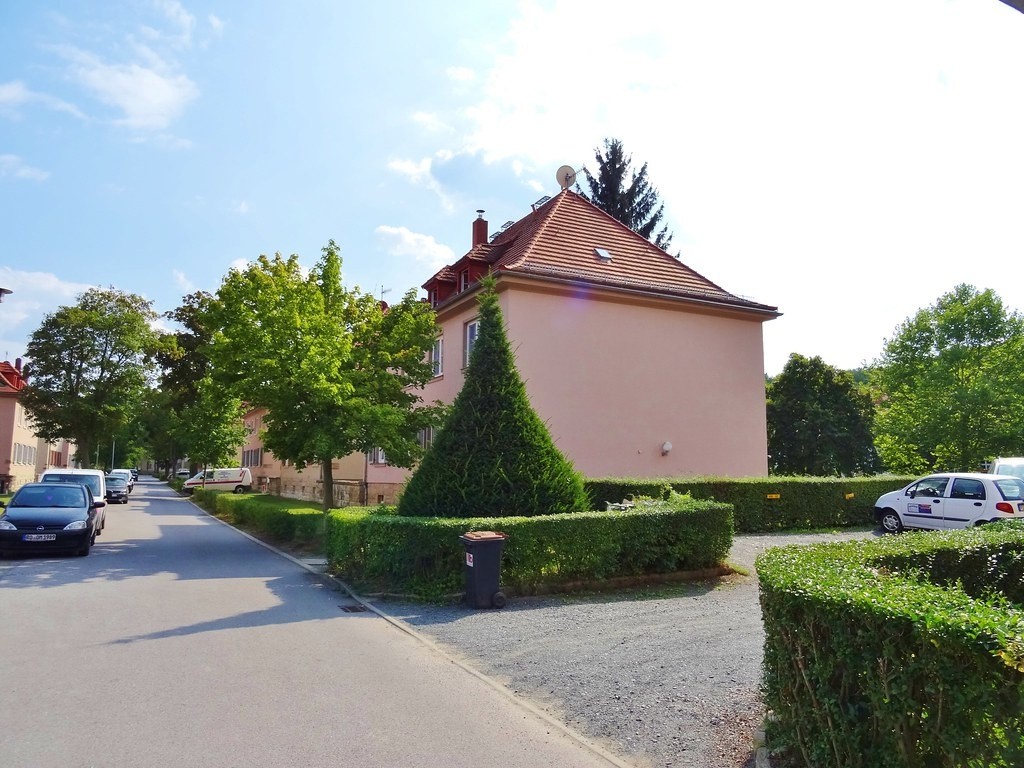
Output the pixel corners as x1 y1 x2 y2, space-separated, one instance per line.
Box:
183 468 253 494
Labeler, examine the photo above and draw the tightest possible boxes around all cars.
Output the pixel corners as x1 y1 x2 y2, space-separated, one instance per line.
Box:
40 469 107 535
105 469 133 493
0 481 105 556
105 474 128 503
130 469 138 482
874 473 1024 533
167 471 190 479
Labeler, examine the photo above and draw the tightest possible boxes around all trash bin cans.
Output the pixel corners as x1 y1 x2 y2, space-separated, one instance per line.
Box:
459 529 508 609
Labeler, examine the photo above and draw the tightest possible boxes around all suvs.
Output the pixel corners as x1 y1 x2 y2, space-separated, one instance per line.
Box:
988 456 1024 482
